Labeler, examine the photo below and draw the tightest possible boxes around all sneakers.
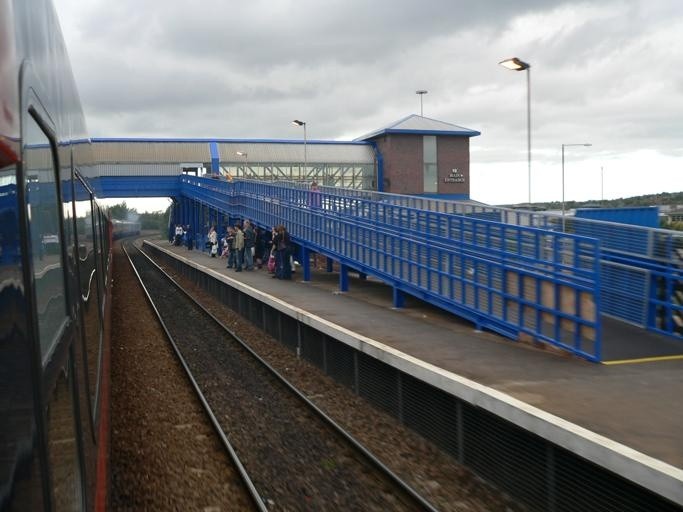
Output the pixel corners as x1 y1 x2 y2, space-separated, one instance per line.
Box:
226 265 254 272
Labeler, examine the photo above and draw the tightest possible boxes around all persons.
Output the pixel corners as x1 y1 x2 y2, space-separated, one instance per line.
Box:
210 172 219 192
305 181 321 233
223 174 233 195
171 219 296 280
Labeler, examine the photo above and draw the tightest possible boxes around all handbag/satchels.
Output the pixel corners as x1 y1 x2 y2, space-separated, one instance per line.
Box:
212 244 217 255
267 254 277 274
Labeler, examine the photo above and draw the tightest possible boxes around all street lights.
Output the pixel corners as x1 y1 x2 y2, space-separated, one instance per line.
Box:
416 90 427 115
498 58 532 205
292 120 307 182
237 152 247 177
562 144 592 232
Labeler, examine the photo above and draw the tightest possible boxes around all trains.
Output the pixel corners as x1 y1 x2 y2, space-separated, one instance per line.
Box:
0 0 142 511
